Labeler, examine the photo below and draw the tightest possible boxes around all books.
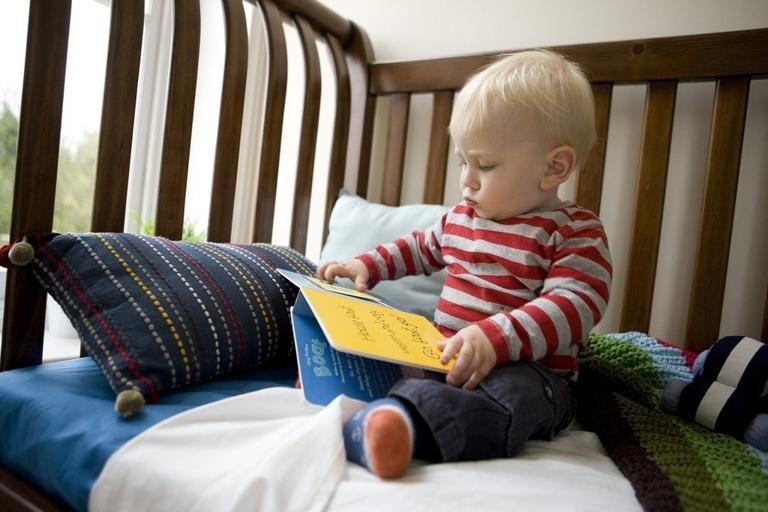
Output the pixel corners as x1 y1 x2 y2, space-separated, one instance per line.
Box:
275 267 460 410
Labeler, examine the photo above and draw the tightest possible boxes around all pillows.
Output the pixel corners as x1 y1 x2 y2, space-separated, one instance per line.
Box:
317 187 451 324
0 230 340 418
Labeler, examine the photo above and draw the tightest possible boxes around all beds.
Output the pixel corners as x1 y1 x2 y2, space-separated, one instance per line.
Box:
0 0 768 512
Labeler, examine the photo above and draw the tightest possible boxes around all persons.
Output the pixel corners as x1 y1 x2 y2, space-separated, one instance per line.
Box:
294 48 614 480
